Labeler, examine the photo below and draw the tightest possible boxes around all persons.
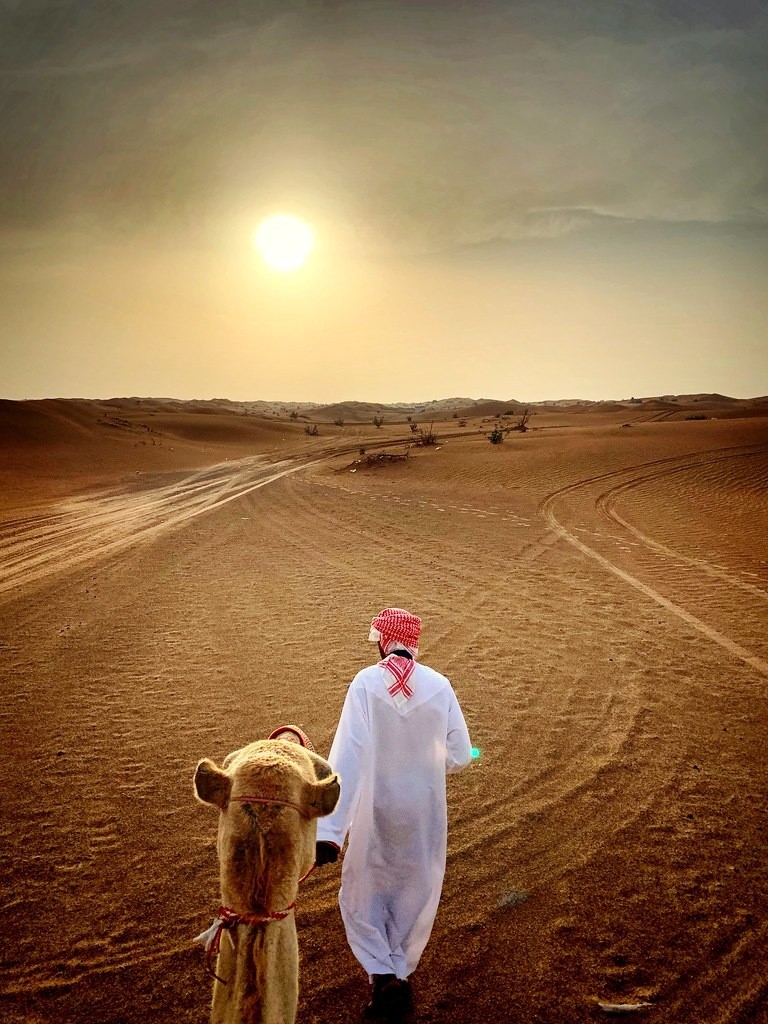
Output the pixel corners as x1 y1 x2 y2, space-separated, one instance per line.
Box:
316 606 472 1024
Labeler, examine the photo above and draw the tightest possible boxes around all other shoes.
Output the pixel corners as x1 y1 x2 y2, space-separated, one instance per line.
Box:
364 974 413 1024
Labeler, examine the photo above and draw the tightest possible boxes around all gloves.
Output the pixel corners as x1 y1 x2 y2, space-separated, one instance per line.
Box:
316 841 338 868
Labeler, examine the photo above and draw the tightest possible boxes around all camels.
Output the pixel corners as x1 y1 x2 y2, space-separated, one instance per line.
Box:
191 725 341 1024
